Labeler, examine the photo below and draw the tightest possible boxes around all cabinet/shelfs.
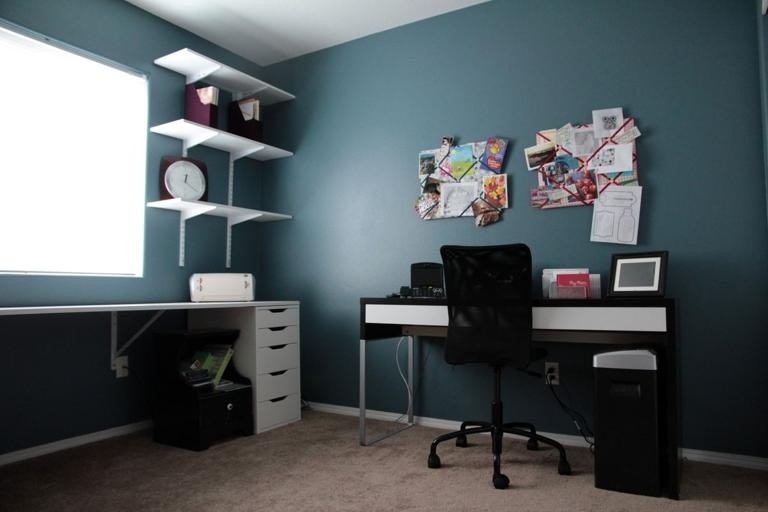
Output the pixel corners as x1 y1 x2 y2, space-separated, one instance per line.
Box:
146 46 297 269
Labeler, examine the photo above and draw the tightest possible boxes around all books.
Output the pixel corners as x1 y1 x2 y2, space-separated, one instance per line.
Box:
195 86 260 121
175 344 252 392
541 267 601 298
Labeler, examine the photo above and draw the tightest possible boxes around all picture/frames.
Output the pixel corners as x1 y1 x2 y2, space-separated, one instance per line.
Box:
605 249 671 299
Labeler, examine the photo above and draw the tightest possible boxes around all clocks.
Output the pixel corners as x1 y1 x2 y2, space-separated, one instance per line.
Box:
159 155 211 203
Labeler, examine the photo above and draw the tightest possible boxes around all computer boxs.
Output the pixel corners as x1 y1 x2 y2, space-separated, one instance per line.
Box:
595 350 663 498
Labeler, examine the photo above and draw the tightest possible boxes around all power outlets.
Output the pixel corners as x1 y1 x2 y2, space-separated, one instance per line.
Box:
543 361 561 385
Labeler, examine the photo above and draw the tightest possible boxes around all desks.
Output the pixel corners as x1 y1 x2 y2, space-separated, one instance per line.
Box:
356 294 689 502
0 299 303 434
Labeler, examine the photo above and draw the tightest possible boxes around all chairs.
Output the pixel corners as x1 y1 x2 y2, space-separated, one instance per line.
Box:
426 242 573 491
